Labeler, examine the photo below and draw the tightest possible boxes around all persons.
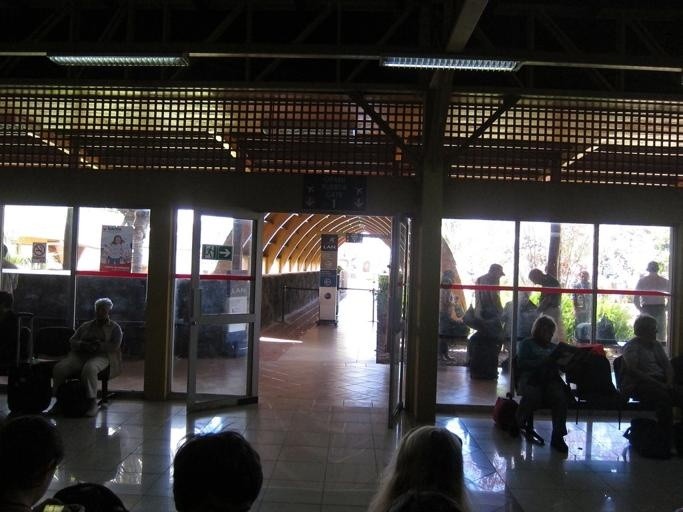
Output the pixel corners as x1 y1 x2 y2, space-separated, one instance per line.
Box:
0 416 81 510
56 482 130 512
368 426 475 512
102 235 133 264
0 244 18 310
173 430 258 512
387 490 462 512
49 298 123 418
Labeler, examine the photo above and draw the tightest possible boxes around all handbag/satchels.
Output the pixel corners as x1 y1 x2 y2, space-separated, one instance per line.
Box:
56 382 90 417
576 359 625 409
493 392 521 434
623 416 667 460
597 312 618 344
462 302 480 331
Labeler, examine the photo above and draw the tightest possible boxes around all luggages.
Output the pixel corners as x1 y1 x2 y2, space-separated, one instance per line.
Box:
468 331 499 380
5 362 52 414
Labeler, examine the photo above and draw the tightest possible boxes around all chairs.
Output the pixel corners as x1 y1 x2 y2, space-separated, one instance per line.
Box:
514 346 681 433
0 316 123 417
